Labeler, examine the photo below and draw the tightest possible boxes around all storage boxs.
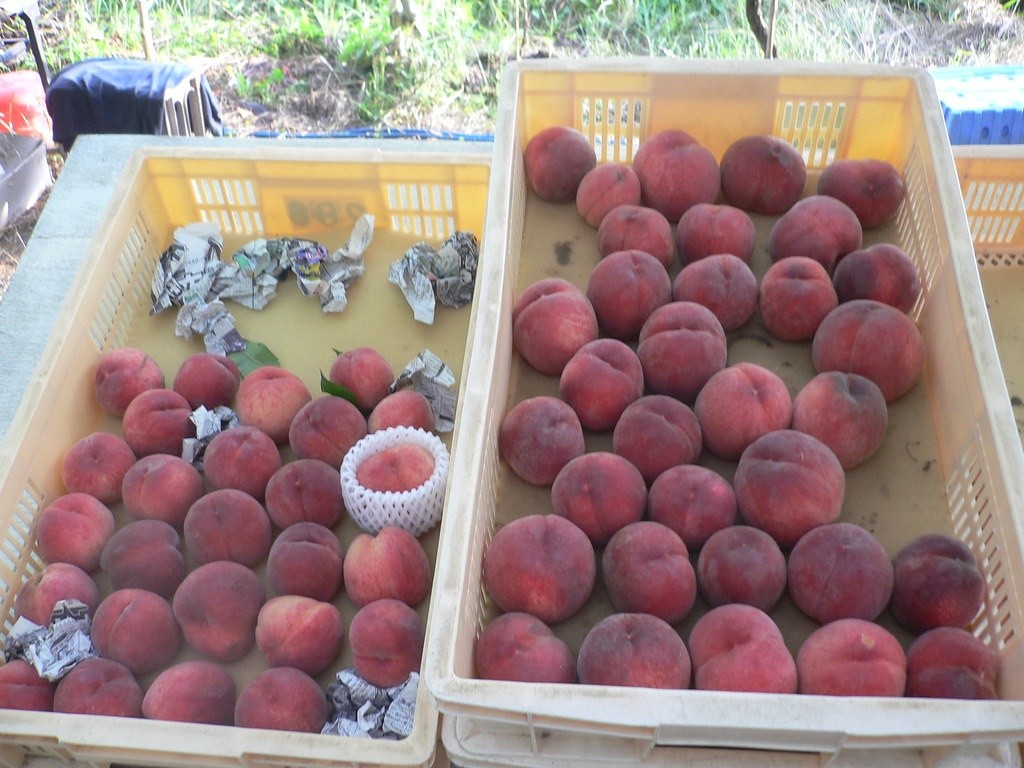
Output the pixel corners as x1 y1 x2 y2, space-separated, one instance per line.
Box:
0 58 1023 767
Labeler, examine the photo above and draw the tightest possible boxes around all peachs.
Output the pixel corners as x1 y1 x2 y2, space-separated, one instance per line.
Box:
477 121 1000 701
0 347 436 731
